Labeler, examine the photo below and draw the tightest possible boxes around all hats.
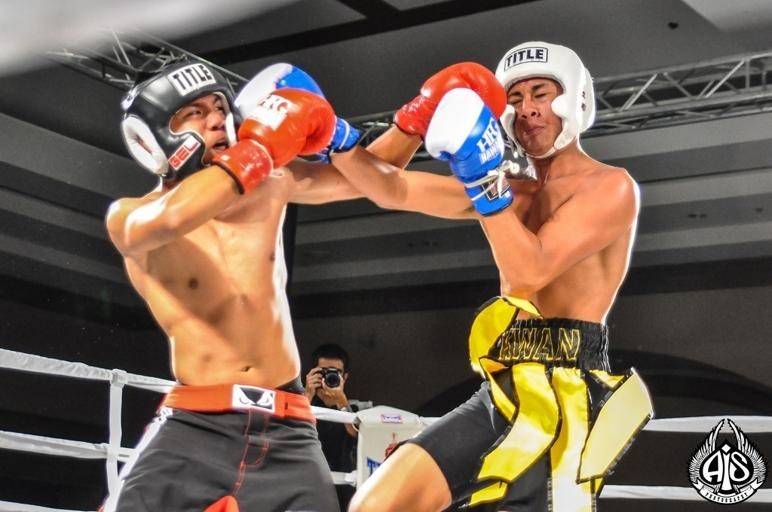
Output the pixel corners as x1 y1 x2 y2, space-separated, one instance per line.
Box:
117 59 245 190
495 39 598 160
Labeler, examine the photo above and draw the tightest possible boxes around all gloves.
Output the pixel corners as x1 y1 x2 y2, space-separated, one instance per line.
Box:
208 59 363 197
390 59 514 216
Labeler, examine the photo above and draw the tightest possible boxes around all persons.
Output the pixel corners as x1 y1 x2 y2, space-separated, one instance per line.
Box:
98 56 508 512
232 38 658 512
303 342 365 512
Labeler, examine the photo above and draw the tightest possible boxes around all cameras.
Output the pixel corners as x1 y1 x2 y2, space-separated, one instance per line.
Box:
319 367 342 388
